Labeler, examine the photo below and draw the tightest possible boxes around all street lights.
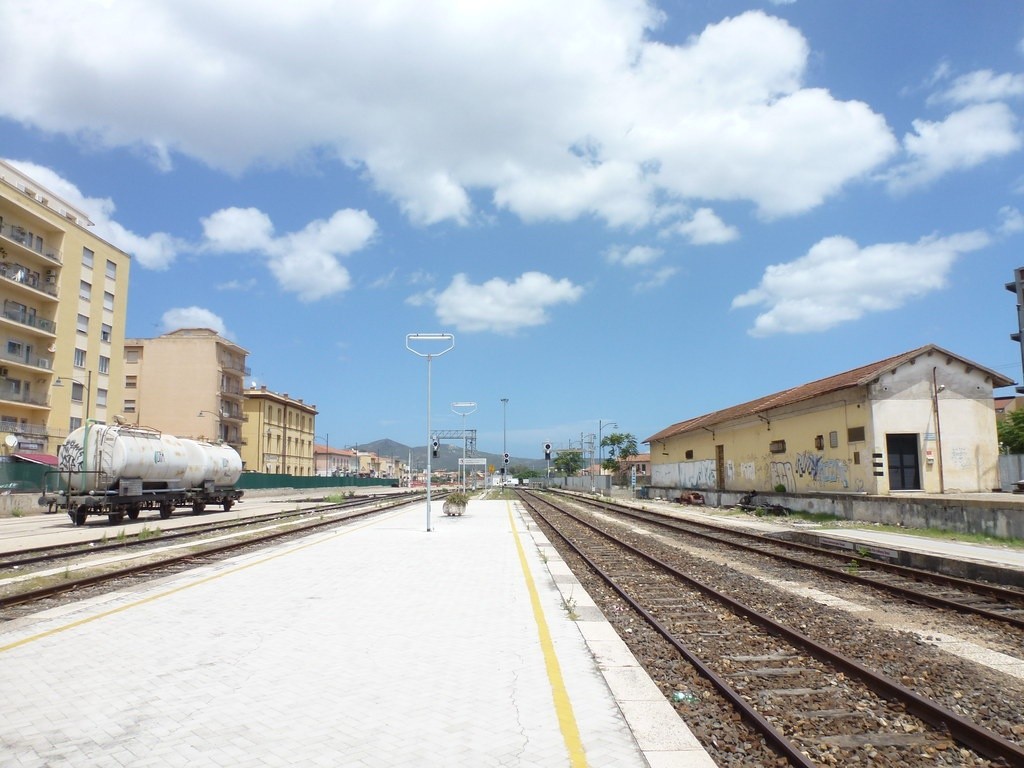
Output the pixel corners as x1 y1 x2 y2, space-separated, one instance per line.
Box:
345 441 358 477
580 432 597 476
313 433 328 477
599 420 618 475
197 406 224 441
935 384 946 494
569 438 582 476
52 371 91 420
500 398 509 481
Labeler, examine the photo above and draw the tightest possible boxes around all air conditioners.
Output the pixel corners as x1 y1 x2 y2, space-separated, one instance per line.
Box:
46 269 55 276
36 358 49 369
0 367 9 376
46 276 56 284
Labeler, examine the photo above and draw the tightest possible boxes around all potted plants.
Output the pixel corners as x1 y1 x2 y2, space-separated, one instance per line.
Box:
442 492 471 516
